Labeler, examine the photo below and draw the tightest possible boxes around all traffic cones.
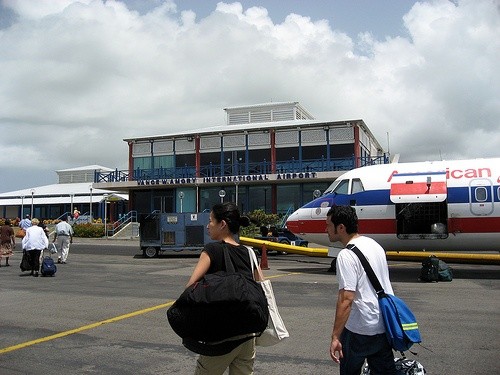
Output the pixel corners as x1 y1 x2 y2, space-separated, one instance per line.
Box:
260 243 270 271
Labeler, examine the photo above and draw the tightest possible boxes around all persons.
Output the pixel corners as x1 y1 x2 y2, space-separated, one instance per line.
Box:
73 207 81 219
52 215 74 264
325 205 402 375
0 219 15 267
260 222 280 237
22 218 48 277
19 214 50 259
186 202 265 375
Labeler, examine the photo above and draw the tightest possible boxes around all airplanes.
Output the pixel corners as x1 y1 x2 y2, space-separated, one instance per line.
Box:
285 158 500 272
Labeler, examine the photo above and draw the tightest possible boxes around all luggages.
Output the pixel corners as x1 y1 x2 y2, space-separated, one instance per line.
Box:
41 247 57 278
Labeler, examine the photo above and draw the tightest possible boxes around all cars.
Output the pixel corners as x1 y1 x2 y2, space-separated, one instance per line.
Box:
252 227 309 255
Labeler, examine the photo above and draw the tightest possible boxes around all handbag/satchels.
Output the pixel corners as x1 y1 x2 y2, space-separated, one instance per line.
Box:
167 240 270 358
378 290 425 356
244 244 289 350
361 355 426 375
15 228 25 237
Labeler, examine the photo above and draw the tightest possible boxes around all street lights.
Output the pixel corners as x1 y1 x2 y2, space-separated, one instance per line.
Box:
178 192 185 213
219 189 226 204
233 176 241 208
103 194 108 236
88 182 95 223
386 131 390 163
29 188 36 221
70 191 75 215
313 189 322 199
19 193 25 220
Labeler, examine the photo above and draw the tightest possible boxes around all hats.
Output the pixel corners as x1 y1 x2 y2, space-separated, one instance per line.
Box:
4 219 13 224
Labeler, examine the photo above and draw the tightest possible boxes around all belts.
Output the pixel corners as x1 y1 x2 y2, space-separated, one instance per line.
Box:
59 234 68 236
